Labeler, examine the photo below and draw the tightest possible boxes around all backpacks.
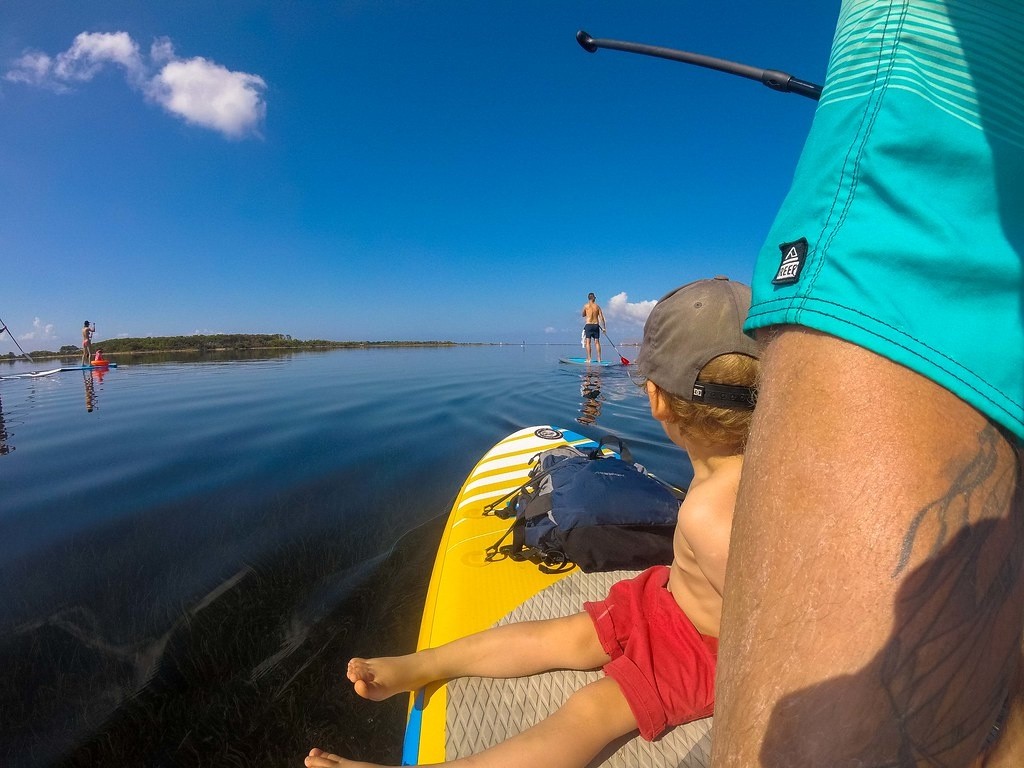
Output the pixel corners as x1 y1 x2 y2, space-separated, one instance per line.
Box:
495 435 682 574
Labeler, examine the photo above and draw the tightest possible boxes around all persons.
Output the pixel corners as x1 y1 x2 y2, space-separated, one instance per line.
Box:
302 273 760 768
95 347 104 360
707 2 1024 768
582 293 607 362
81 320 95 367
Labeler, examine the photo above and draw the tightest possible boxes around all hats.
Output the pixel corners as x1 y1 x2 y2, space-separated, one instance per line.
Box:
638 276 770 409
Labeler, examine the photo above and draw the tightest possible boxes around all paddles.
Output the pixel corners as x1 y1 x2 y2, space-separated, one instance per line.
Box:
571 30 825 101
0 318 35 364
598 324 630 366
86 322 96 358
